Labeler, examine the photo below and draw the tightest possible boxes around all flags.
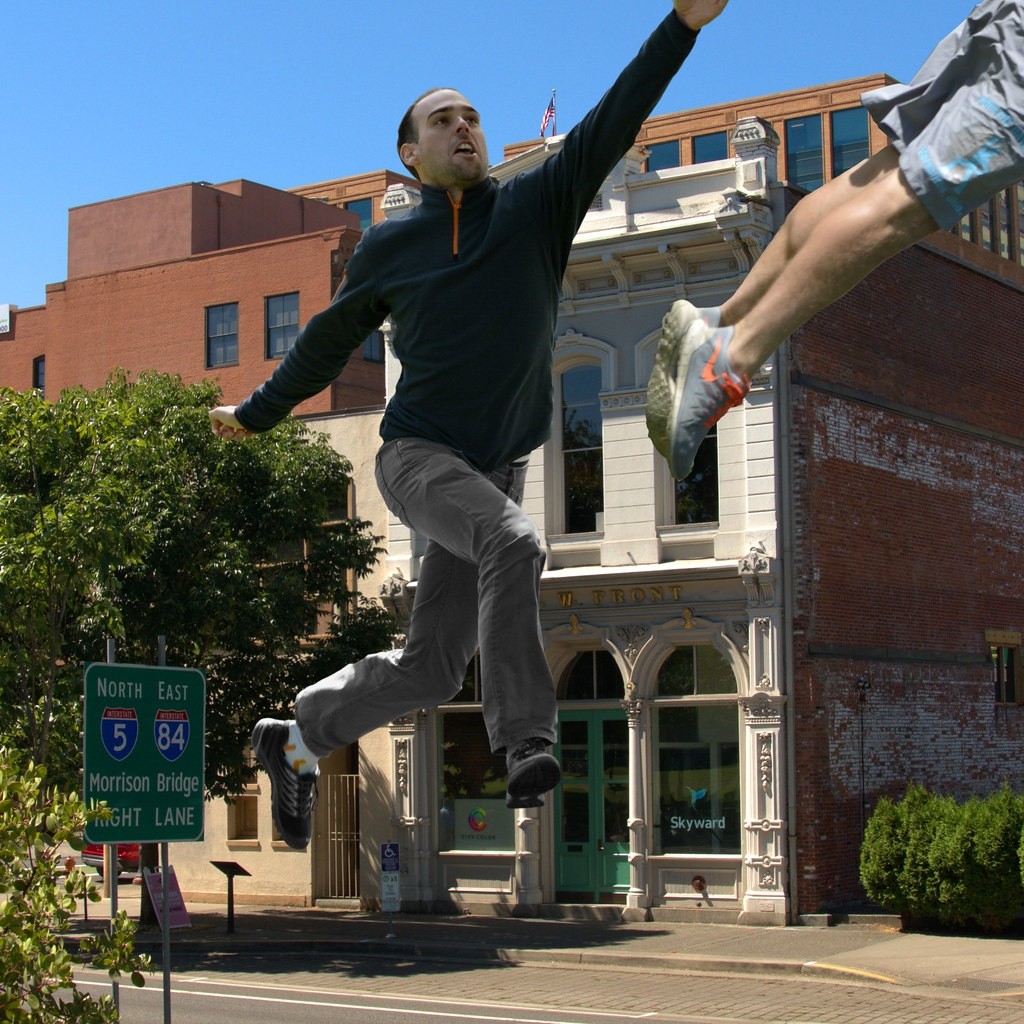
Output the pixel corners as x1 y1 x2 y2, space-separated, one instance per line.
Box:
540 98 555 138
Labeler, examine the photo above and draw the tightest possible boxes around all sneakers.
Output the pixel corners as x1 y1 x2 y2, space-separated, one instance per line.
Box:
251 718 321 850
505 738 560 809
646 299 750 482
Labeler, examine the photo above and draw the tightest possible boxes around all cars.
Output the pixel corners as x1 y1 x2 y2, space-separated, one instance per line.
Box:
82 840 139 880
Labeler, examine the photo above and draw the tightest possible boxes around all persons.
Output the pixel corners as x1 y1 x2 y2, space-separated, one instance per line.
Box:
646 2 1024 481
209 1 726 850
